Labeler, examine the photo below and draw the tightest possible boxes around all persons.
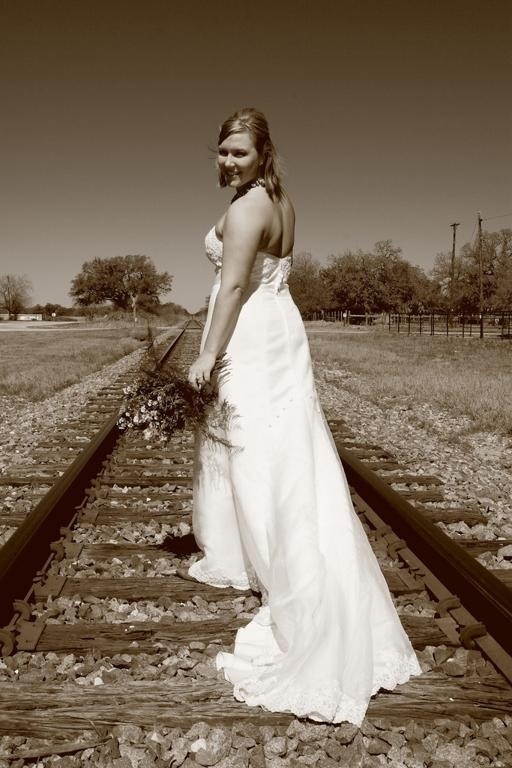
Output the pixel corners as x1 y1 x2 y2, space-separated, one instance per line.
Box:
183 104 425 731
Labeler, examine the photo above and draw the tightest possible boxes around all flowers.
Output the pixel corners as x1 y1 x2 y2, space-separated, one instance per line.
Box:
116 375 198 444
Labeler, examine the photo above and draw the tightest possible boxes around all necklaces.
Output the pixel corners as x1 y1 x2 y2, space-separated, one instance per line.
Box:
236 175 260 199
